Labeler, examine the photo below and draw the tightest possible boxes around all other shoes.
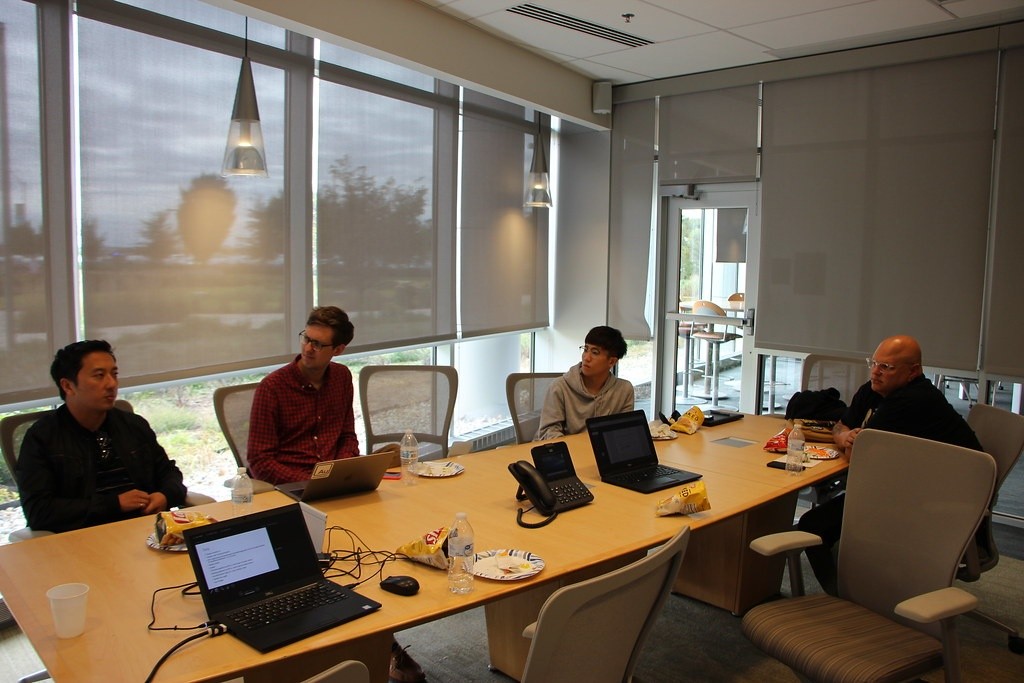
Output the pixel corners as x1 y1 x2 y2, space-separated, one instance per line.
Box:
389 644 425 683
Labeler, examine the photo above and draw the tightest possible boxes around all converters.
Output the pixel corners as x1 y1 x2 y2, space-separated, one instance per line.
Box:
317 553 332 568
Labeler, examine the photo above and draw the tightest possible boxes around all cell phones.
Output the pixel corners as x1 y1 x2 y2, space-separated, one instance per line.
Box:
767 461 806 472
383 471 402 480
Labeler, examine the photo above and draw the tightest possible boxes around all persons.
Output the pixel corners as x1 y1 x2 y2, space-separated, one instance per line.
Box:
246 306 425 683
17 340 186 534
533 326 635 443
799 335 984 598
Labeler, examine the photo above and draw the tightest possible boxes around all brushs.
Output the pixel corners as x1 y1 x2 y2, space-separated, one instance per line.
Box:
860 408 878 429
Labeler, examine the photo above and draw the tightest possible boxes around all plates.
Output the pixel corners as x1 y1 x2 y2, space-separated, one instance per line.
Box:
462 549 547 581
804 446 840 459
652 430 678 440
407 461 465 478
145 532 188 551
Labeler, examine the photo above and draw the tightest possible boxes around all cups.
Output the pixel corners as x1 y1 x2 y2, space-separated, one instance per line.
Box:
46 582 90 639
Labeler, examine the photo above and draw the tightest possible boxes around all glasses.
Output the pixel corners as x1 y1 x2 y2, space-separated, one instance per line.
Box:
579 346 608 356
299 330 338 351
866 358 894 371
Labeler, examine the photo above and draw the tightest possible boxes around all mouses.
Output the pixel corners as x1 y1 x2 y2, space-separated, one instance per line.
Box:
380 576 420 596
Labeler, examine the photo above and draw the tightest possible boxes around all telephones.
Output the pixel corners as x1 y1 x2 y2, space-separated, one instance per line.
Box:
507 441 594 517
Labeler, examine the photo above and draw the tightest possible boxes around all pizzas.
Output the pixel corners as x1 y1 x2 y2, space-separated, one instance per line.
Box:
805 447 830 458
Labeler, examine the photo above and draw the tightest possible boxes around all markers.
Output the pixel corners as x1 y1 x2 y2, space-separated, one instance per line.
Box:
710 410 730 416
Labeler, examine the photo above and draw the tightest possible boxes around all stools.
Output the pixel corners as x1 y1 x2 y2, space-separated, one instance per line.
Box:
673 291 751 412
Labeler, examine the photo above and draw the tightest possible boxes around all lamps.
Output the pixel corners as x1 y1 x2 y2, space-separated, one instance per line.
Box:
221 14 269 179
522 109 553 206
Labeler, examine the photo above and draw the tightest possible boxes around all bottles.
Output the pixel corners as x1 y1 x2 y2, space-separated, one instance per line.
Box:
400 429 419 486
231 467 254 518
785 424 805 476
447 511 475 596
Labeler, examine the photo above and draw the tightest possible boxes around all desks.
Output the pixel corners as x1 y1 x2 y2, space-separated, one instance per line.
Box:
0 406 857 683
673 296 780 414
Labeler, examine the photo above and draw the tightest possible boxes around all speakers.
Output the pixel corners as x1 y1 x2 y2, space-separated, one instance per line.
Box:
593 82 611 114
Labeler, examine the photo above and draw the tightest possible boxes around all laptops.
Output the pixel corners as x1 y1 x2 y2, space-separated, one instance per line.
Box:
274 451 395 503
585 409 703 494
701 410 743 428
182 504 382 654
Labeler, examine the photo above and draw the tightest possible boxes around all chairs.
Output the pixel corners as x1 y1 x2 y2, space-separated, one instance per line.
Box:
917 402 1021 654
214 380 261 480
506 372 565 447
797 353 876 413
0 409 56 531
744 428 998 681
359 366 460 459
518 523 691 682
